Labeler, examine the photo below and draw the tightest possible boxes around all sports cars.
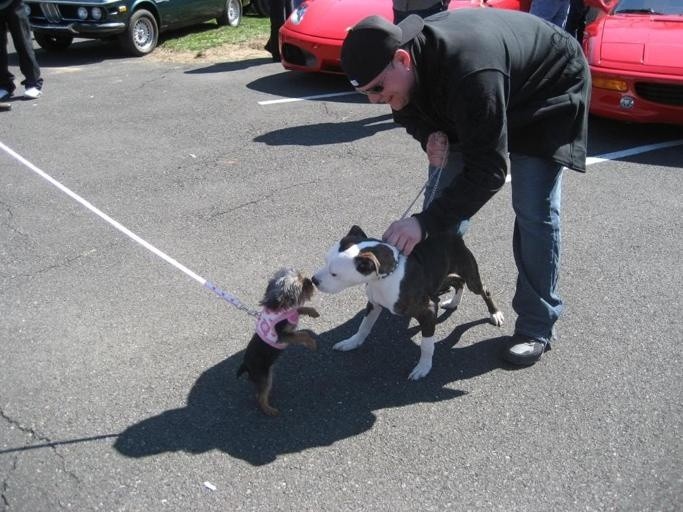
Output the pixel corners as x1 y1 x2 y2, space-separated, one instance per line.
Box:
277 1 533 78
581 1 683 126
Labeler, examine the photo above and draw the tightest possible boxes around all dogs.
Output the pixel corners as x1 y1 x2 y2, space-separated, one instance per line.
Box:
312 223 506 381
235 265 321 418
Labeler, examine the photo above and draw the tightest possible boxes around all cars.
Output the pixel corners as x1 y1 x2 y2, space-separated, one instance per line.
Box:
21 0 251 56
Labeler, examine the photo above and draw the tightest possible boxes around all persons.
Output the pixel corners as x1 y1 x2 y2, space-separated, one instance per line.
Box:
341 8 593 365
0 0 43 100
264 0 294 62
392 0 590 36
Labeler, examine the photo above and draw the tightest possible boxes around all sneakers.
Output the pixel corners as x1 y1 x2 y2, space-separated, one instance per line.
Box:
23 87 42 99
0 89 11 112
502 332 546 366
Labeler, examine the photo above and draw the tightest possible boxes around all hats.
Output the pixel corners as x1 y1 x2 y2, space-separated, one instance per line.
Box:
341 13 425 88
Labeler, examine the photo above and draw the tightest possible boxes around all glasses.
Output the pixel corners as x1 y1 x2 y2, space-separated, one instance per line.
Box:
362 80 384 94
